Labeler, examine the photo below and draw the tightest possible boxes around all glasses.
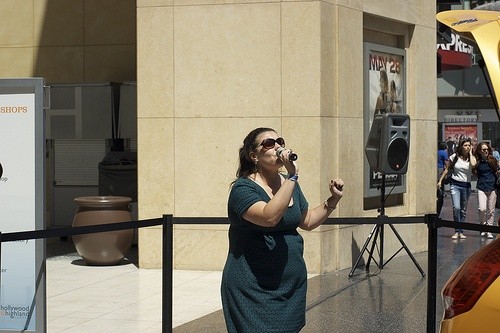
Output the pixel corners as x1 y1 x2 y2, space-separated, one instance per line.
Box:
258 137 285 149
482 149 490 152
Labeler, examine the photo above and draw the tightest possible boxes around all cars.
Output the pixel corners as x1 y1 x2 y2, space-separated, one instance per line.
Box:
436 10 500 333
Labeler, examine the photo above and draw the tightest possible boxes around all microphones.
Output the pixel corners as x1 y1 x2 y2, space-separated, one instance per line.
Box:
276 148 298 161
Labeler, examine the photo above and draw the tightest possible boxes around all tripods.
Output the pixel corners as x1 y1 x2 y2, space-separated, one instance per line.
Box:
348 174 426 277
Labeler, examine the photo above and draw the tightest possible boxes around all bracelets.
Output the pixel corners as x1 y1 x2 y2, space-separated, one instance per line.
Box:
286 173 298 181
324 199 336 210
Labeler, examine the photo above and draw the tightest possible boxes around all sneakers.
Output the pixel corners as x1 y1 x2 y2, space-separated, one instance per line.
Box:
451 232 466 239
480 231 493 239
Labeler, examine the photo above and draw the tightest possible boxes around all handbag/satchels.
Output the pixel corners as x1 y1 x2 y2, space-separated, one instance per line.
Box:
495 169 500 185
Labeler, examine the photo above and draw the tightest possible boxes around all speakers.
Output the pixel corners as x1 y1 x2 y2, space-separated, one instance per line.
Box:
365 114 409 174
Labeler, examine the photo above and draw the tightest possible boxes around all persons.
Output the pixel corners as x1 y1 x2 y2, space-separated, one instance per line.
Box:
437 143 449 182
491 145 500 168
438 134 466 156
472 142 500 238
221 127 344 333
437 137 477 239
375 71 403 115
